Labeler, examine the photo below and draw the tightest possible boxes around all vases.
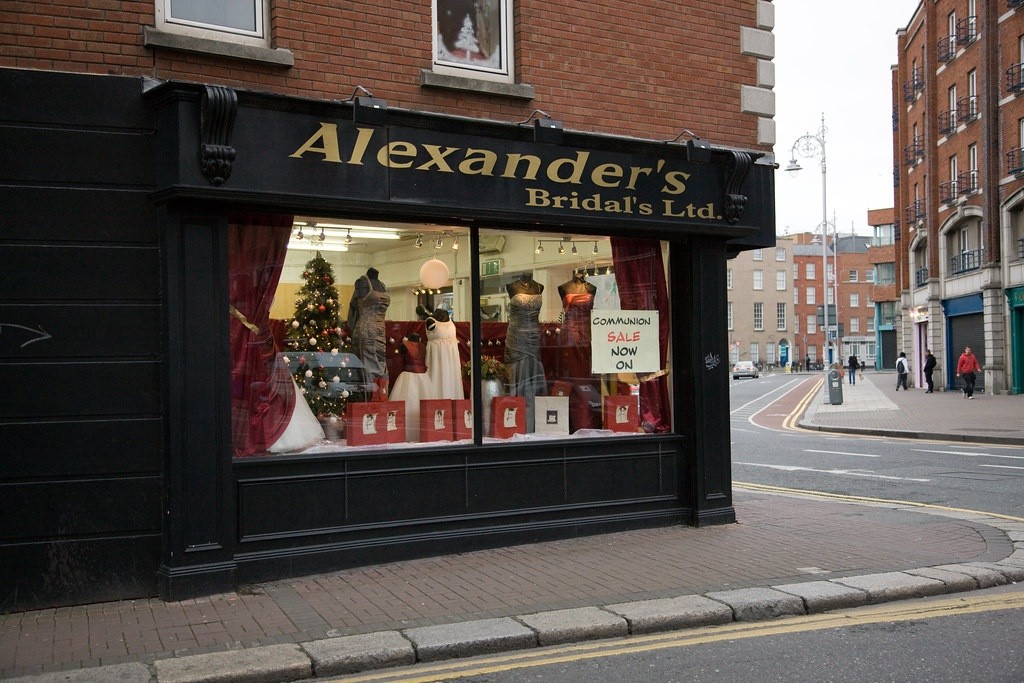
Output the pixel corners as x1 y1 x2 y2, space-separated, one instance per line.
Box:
469 378 506 437
320 416 341 441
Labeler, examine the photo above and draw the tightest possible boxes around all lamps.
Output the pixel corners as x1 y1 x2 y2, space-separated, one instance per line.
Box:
557 242 566 255
515 110 563 144
594 264 599 276
287 241 350 252
571 242 578 255
435 235 443 248
341 86 388 127
592 242 599 254
606 263 612 275
661 130 711 163
437 288 441 294
291 225 401 240
345 229 351 243
452 236 459 250
410 286 434 296
297 226 304 240
413 232 423 248
535 241 544 254
583 266 590 278
318 228 326 241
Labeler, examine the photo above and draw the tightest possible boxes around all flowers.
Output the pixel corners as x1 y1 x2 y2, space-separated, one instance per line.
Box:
462 354 509 381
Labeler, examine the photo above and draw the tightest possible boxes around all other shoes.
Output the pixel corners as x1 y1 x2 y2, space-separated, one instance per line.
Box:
962 390 966 398
968 397 973 400
926 390 933 394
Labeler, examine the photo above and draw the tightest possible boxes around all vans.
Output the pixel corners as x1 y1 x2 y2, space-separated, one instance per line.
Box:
272 352 375 409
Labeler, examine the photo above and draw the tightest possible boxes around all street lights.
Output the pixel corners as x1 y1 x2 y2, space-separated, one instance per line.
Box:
783 111 833 404
810 208 840 363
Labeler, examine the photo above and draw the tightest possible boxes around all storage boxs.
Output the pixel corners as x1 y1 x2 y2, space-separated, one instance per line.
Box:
381 400 407 443
451 400 472 441
489 396 527 440
534 396 569 435
552 381 575 396
347 403 382 446
420 400 454 442
603 395 638 433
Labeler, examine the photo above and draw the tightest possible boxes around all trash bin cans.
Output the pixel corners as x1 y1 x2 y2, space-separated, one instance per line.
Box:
828 368 844 405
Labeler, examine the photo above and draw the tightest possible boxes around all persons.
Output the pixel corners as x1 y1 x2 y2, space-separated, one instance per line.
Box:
423 308 465 399
957 347 981 399
895 352 909 391
390 331 436 442
806 354 809 372
346 268 391 401
505 271 549 433
924 349 933 393
848 356 855 384
557 274 597 430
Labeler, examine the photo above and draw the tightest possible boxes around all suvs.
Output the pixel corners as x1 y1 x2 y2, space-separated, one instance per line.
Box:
508 374 632 432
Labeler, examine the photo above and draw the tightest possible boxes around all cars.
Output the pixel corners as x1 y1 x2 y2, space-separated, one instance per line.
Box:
733 361 759 380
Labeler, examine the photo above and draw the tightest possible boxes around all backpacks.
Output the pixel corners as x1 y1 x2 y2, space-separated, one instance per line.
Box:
930 356 937 368
896 360 905 373
